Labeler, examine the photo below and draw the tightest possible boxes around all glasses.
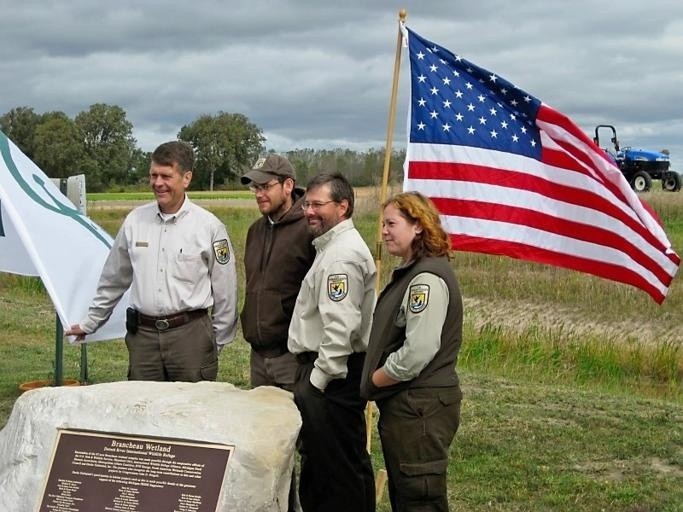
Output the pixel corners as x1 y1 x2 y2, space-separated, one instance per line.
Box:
248 180 283 194
300 199 342 211
137 306 209 330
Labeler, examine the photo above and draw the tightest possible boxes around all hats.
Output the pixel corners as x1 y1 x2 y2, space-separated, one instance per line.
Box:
238 154 297 186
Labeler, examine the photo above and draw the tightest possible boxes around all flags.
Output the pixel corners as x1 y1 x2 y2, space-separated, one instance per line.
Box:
0 129 133 345
399 20 682 305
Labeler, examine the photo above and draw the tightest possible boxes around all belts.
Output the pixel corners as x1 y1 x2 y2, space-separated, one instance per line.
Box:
294 350 321 366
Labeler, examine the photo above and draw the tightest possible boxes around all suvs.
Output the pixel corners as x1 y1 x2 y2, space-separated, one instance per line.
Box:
593 124 682 192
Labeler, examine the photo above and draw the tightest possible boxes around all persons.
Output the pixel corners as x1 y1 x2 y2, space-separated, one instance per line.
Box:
287 172 378 512
240 153 317 512
66 141 239 383
360 191 463 512
